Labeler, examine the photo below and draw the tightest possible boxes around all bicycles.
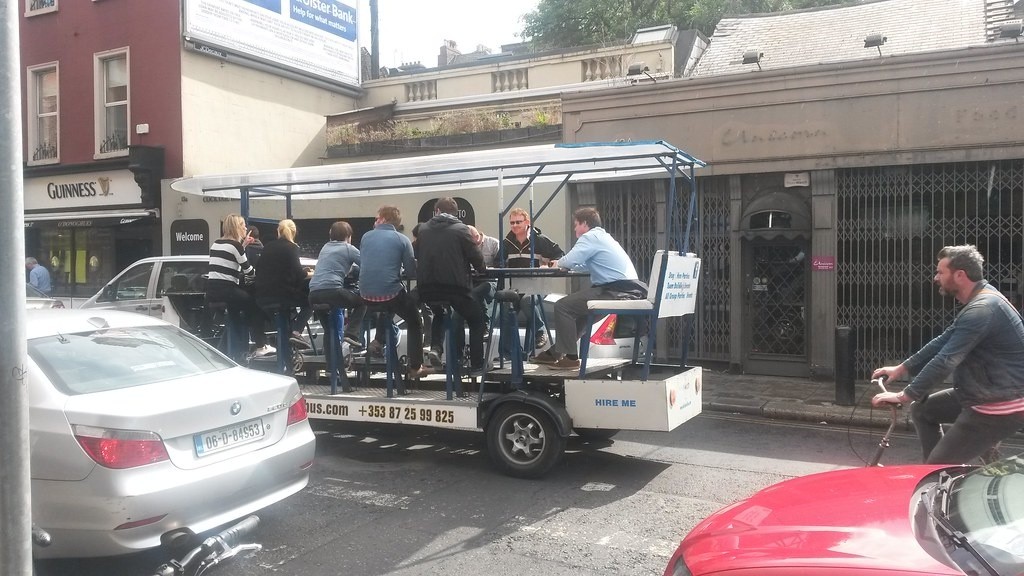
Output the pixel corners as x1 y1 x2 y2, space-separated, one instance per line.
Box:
846 375 902 468
153 514 264 576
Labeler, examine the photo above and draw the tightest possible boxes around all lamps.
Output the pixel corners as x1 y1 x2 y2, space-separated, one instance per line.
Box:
50 255 60 272
742 51 764 70
1000 22 1024 42
865 33 887 55
88 254 100 274
627 62 656 84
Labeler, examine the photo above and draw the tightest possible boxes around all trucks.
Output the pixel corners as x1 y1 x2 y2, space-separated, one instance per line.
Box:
77 139 706 480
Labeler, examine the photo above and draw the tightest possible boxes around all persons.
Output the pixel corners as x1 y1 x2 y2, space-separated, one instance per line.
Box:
357 205 429 378
203 215 268 347
527 204 645 370
417 196 495 376
244 226 268 270
872 244 1024 465
26 256 51 295
493 207 564 362
253 218 313 376
393 222 500 348
307 221 366 376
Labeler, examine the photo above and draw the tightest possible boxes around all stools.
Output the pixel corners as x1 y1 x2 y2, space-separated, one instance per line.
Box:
203 277 573 399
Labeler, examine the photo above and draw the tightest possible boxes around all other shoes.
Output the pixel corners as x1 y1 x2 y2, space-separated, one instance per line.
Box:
394 328 402 347
289 334 310 350
256 345 277 357
535 329 549 348
469 361 494 377
483 323 490 340
410 366 427 379
343 333 362 347
422 346 431 353
369 341 384 360
428 350 443 372
325 369 332 376
494 353 512 362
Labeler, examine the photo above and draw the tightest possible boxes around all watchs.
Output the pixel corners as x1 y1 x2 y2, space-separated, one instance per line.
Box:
897 392 906 403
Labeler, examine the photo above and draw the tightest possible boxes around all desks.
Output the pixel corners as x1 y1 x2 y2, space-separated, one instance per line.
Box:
236 265 577 369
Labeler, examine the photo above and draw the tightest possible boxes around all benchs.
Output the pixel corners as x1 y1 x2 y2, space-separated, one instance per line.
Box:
576 250 705 378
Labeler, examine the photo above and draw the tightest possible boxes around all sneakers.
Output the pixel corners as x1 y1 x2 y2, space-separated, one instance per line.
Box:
530 351 556 364
547 354 580 369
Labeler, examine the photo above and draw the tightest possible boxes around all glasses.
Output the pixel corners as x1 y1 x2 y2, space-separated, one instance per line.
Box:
510 220 526 225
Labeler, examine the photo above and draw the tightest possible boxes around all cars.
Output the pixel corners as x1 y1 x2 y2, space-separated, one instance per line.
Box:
32 309 317 561
664 451 1023 576
